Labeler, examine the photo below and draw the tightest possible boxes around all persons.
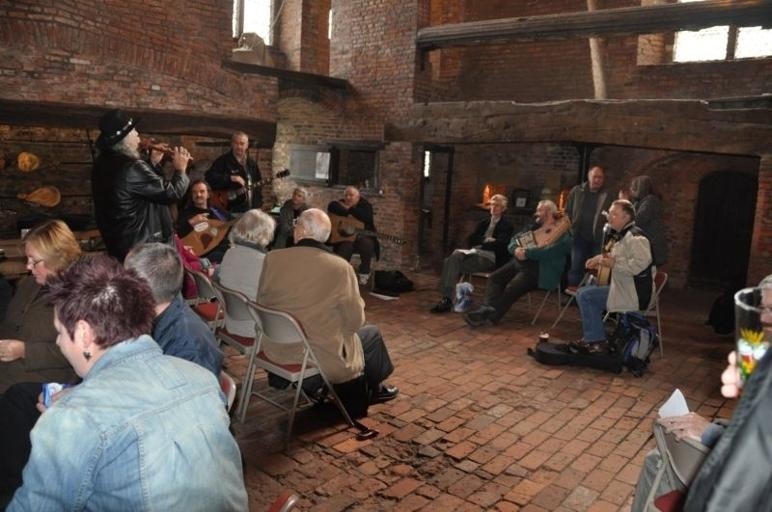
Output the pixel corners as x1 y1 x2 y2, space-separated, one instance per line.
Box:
175 130 399 404
627 273 772 511
2 255 249 511
430 167 667 356
91 107 191 264
2 219 223 511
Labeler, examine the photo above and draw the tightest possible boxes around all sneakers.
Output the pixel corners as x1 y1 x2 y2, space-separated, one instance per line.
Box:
568 338 601 354
358 273 371 285
463 305 497 327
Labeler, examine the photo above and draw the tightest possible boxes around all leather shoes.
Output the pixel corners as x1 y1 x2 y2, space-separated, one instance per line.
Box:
370 385 399 405
430 296 452 313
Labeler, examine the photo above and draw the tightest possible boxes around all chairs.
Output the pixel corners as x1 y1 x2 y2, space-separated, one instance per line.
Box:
183 263 226 327
643 417 714 512
209 282 308 417
463 242 668 364
242 300 352 440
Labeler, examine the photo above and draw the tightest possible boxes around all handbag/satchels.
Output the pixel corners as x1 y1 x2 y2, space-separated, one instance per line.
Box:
374 270 413 292
613 311 659 377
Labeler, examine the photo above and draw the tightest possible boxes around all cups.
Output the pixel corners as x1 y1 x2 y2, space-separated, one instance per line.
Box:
733 285 772 397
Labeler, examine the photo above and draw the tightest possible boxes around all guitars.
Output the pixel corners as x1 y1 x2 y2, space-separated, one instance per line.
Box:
207 169 290 210
329 212 406 245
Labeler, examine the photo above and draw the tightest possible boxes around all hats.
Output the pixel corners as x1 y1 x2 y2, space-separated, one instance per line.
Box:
95 108 141 148
